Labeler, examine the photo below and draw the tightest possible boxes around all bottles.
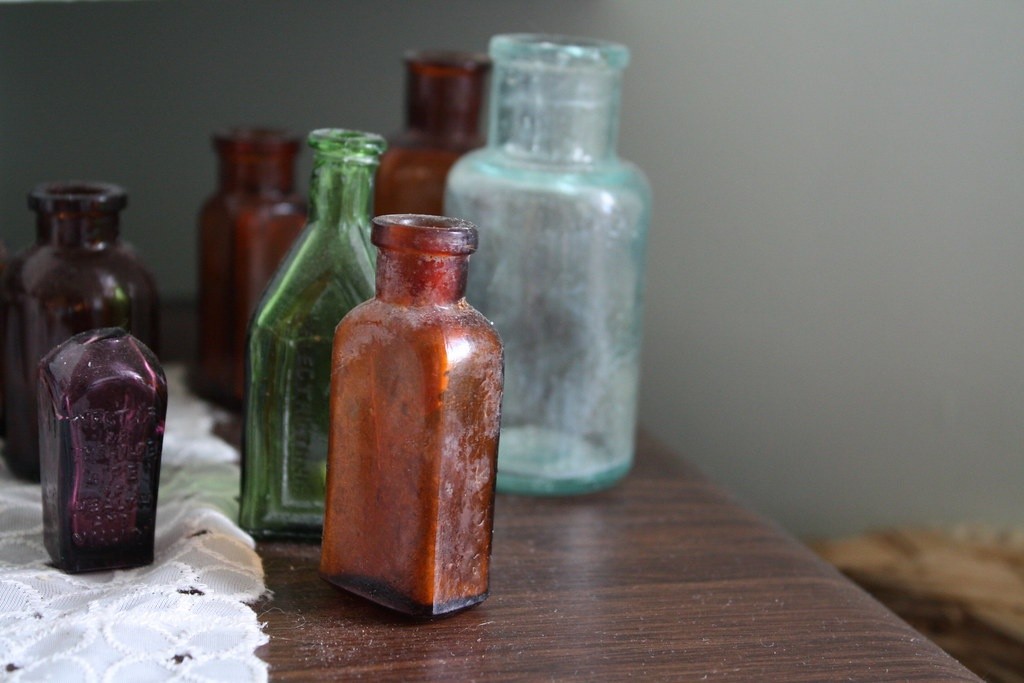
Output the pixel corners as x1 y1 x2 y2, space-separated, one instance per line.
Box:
330 213 504 622
193 126 311 409
0 178 159 479
449 33 655 503
239 130 385 537
41 326 168 575
368 48 493 243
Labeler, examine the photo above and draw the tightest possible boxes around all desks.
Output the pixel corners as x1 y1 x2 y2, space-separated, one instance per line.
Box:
0 296 981 682
